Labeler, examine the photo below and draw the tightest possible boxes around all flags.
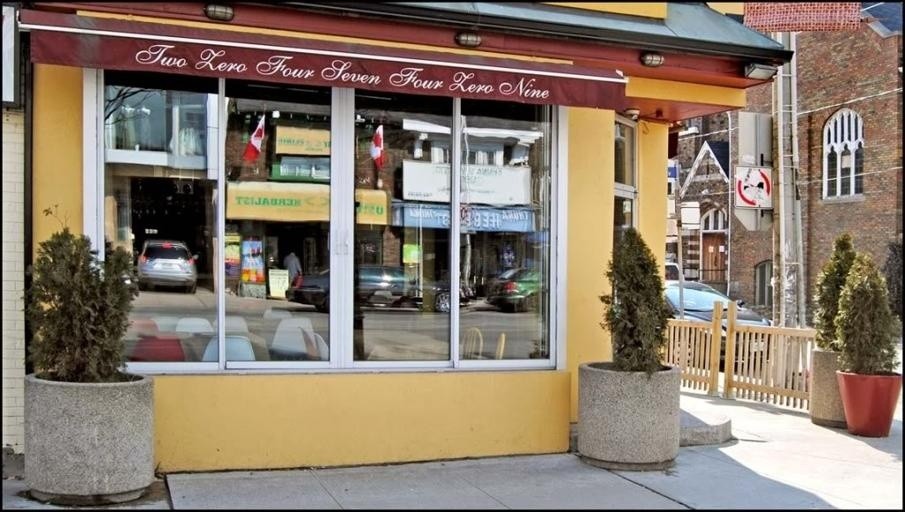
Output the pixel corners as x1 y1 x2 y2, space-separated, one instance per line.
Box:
241 115 266 162
369 124 385 170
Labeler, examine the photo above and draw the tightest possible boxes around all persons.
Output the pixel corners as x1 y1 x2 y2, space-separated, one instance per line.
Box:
283 252 302 281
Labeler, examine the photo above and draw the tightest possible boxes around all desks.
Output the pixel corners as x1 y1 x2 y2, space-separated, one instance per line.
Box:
353 329 450 362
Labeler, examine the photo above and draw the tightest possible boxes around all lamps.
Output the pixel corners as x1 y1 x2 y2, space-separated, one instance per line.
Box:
743 58 780 84
199 2 236 23
638 50 668 69
455 29 481 51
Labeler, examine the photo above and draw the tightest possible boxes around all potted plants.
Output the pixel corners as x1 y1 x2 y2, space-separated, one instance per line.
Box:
806 228 902 437
18 204 157 506
576 224 686 470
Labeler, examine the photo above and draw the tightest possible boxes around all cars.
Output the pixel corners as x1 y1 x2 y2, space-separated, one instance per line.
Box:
138 239 199 294
487 266 549 308
285 265 470 313
666 281 776 367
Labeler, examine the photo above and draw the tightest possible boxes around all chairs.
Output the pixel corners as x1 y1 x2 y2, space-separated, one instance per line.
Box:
462 327 507 360
